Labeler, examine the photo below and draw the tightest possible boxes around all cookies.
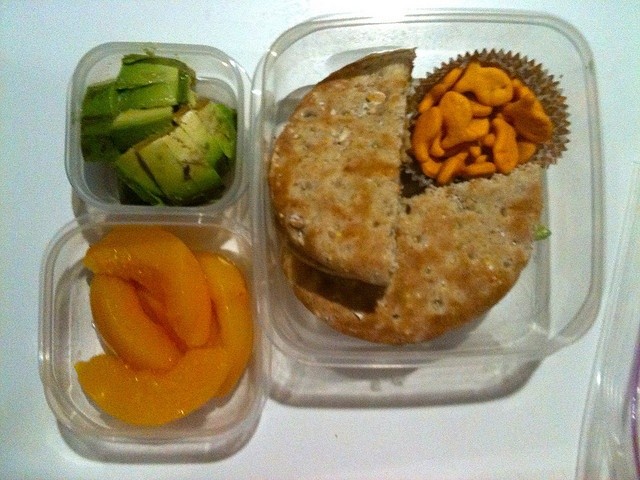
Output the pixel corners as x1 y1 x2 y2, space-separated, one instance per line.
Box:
409 59 555 185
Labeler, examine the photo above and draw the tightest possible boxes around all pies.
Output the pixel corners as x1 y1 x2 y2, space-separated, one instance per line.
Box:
277 162 545 346
264 47 416 286
